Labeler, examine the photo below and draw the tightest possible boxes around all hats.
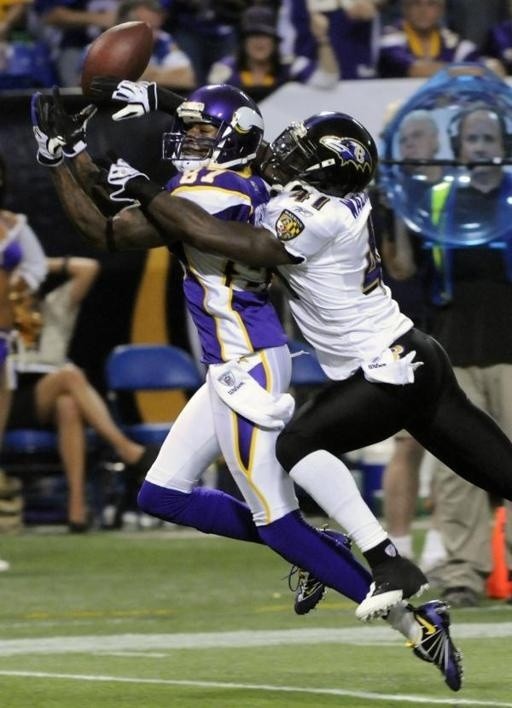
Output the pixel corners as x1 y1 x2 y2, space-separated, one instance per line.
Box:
231 6 284 45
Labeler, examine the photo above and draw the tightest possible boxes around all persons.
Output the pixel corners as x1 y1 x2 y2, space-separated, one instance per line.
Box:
411 110 512 610
379 1 506 77
276 0 382 84
370 110 454 578
6 258 167 535
0 209 47 572
31 84 464 693
1 0 195 84
83 77 512 621
208 5 282 84
488 1 512 73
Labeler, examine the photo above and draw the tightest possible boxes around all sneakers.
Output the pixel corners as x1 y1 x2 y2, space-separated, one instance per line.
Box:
352 536 432 624
404 595 464 693
442 583 478 611
290 528 352 617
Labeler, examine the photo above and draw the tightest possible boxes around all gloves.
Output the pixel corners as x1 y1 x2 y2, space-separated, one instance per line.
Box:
26 88 70 171
84 74 160 124
89 149 168 217
47 85 101 159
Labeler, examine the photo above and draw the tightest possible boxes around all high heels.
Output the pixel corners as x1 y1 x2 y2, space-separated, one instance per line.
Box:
125 448 156 481
67 501 90 533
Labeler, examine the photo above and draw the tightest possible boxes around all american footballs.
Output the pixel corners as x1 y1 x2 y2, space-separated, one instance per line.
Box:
81 20 153 107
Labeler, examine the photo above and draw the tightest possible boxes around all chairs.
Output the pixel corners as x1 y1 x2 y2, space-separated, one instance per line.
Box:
104 342 207 532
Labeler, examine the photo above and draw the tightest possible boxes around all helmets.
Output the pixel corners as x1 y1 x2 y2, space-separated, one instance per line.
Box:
266 108 380 200
155 82 266 175
